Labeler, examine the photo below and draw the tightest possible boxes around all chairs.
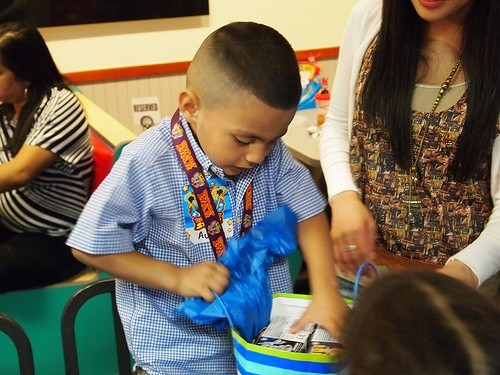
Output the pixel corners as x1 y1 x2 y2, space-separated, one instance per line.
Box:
0 90 139 375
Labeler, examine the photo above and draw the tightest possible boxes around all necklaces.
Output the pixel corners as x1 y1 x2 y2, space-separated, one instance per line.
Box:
408 54 462 220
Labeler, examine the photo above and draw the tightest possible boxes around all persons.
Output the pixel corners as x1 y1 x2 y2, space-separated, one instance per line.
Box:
69 21 357 374
342 269 500 375
0 23 93 292
317 0 500 290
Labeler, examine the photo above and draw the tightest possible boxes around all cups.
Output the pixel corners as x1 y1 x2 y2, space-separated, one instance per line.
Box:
315 94 330 128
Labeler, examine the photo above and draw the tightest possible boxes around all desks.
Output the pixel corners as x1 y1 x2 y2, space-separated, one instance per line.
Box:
283 107 328 188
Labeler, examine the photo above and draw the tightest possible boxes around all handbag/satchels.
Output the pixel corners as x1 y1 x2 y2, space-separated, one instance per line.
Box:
210 259 377 375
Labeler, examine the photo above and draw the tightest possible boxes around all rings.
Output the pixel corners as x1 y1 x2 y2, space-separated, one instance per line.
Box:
343 244 357 254
